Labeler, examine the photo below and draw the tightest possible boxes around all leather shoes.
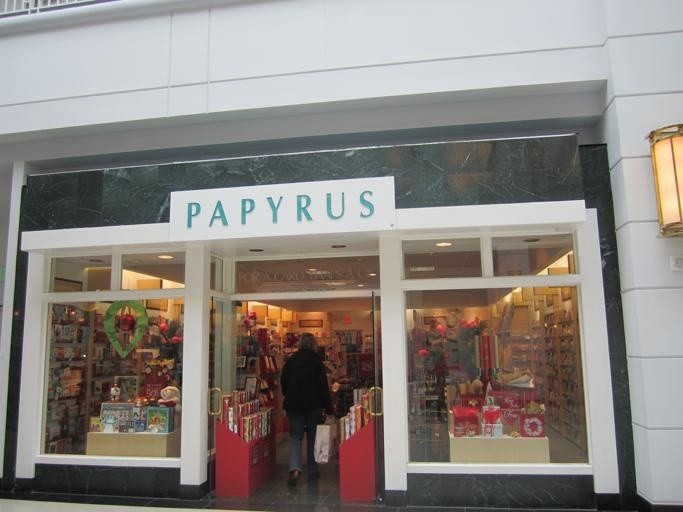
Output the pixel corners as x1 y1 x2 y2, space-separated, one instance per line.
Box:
288 470 300 487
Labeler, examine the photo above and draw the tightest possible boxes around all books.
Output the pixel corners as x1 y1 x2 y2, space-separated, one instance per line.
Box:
49 304 142 454
318 330 375 440
528 309 581 444
222 334 281 444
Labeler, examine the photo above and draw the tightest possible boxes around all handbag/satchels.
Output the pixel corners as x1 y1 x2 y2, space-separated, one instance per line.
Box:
313 417 337 464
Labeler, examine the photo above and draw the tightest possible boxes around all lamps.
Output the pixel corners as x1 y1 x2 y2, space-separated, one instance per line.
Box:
644 122 682 238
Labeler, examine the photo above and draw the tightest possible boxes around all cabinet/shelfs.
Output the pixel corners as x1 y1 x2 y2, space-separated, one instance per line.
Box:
45 285 280 497
337 297 592 463
339 388 377 503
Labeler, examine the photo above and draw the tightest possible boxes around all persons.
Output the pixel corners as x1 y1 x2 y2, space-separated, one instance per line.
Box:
279 332 335 486
